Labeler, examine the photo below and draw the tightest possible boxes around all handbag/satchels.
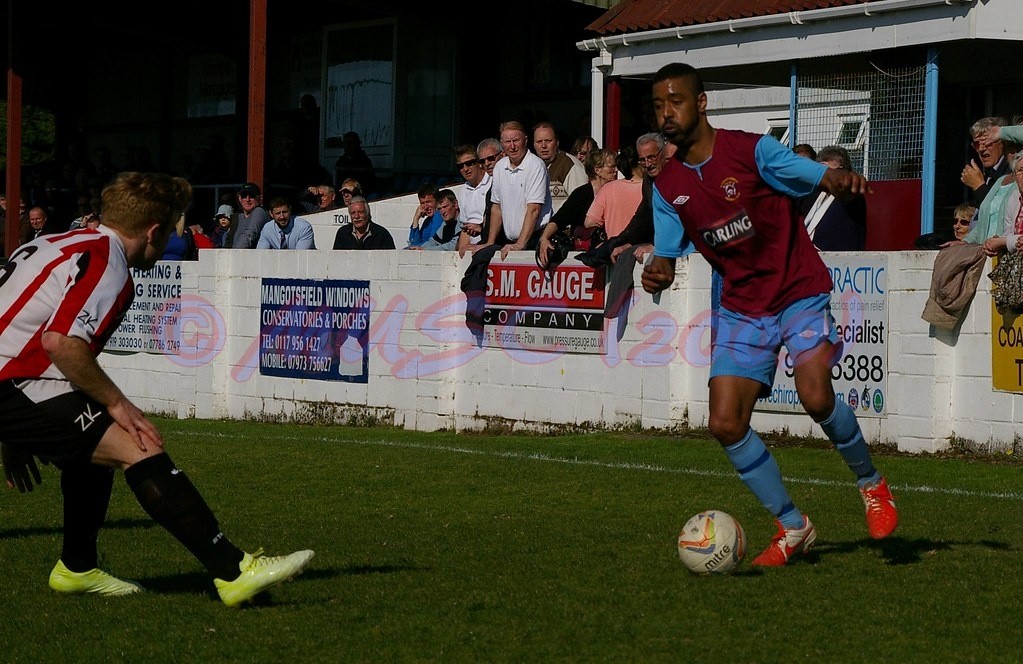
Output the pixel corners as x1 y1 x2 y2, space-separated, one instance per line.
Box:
537 231 572 265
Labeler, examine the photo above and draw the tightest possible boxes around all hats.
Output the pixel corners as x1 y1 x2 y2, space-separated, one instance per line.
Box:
239 183 260 197
215 205 234 218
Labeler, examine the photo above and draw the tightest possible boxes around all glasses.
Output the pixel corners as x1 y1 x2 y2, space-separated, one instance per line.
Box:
972 139 994 150
456 159 479 170
478 150 503 164
637 146 664 164
241 194 257 199
952 218 971 226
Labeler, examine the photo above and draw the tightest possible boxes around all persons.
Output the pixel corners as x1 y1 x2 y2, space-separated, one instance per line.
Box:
641 63 897 563
256 197 315 249
453 122 676 265
336 132 376 199
0 171 317 606
66 134 228 183
213 182 267 249
0 194 100 243
939 117 1023 254
161 213 214 260
333 195 396 250
791 143 866 251
403 184 444 250
283 94 320 168
410 190 460 251
339 178 362 207
298 184 337 212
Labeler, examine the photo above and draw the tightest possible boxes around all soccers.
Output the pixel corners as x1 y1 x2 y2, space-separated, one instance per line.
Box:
678 510 748 577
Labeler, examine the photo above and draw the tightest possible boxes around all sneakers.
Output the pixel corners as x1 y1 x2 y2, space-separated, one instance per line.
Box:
858 477 897 539
751 514 817 567
213 547 316 608
49 558 139 597
